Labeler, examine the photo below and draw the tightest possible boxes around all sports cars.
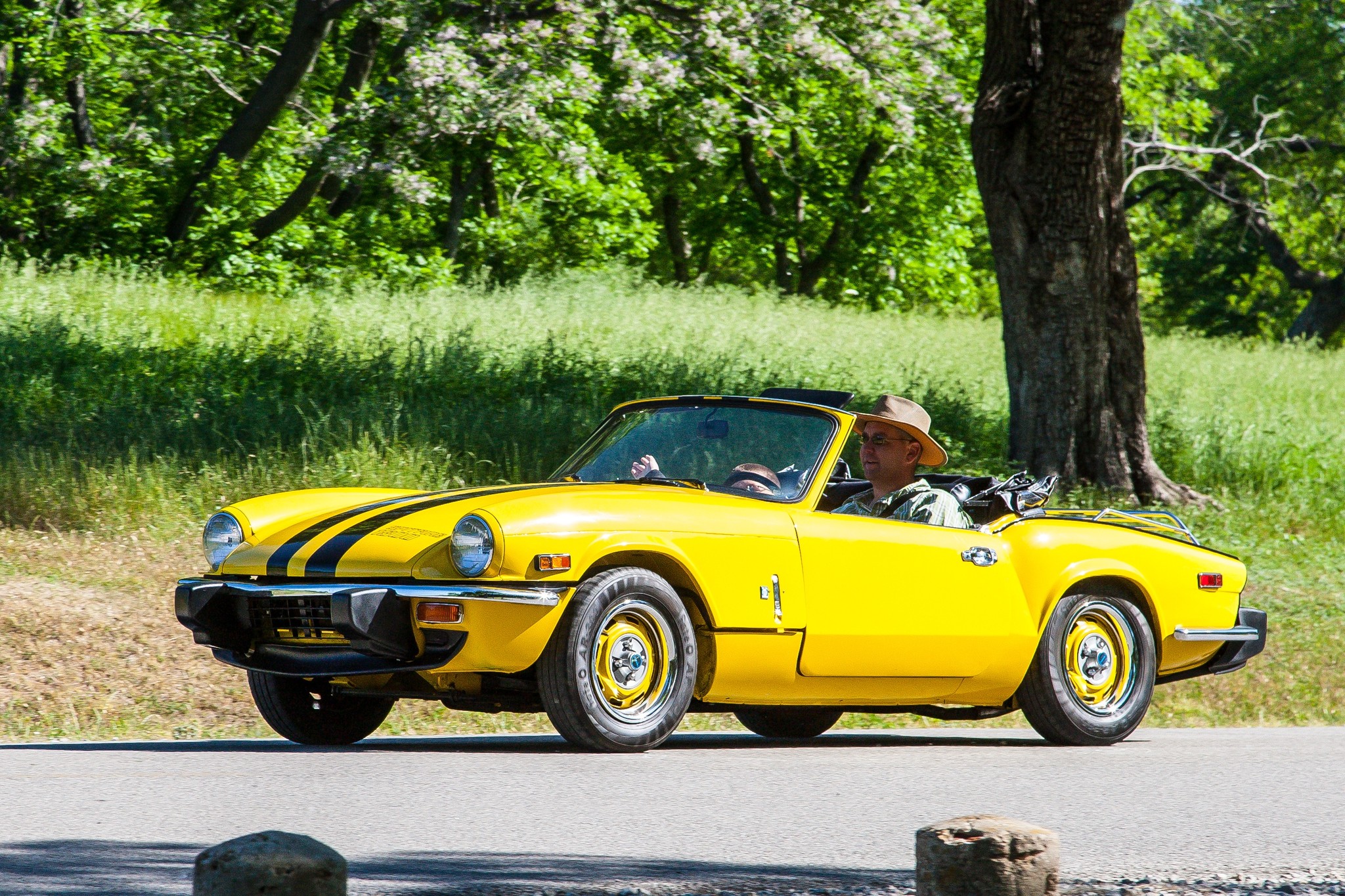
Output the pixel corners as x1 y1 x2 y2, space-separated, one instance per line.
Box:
174 384 1268 753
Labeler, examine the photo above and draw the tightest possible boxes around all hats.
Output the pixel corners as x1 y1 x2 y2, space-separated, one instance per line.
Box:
850 394 948 466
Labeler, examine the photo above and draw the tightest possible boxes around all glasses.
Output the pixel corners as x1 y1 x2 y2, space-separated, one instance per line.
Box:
858 432 913 446
744 484 773 494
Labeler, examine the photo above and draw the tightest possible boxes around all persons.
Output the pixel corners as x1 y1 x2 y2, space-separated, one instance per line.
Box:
631 394 974 528
